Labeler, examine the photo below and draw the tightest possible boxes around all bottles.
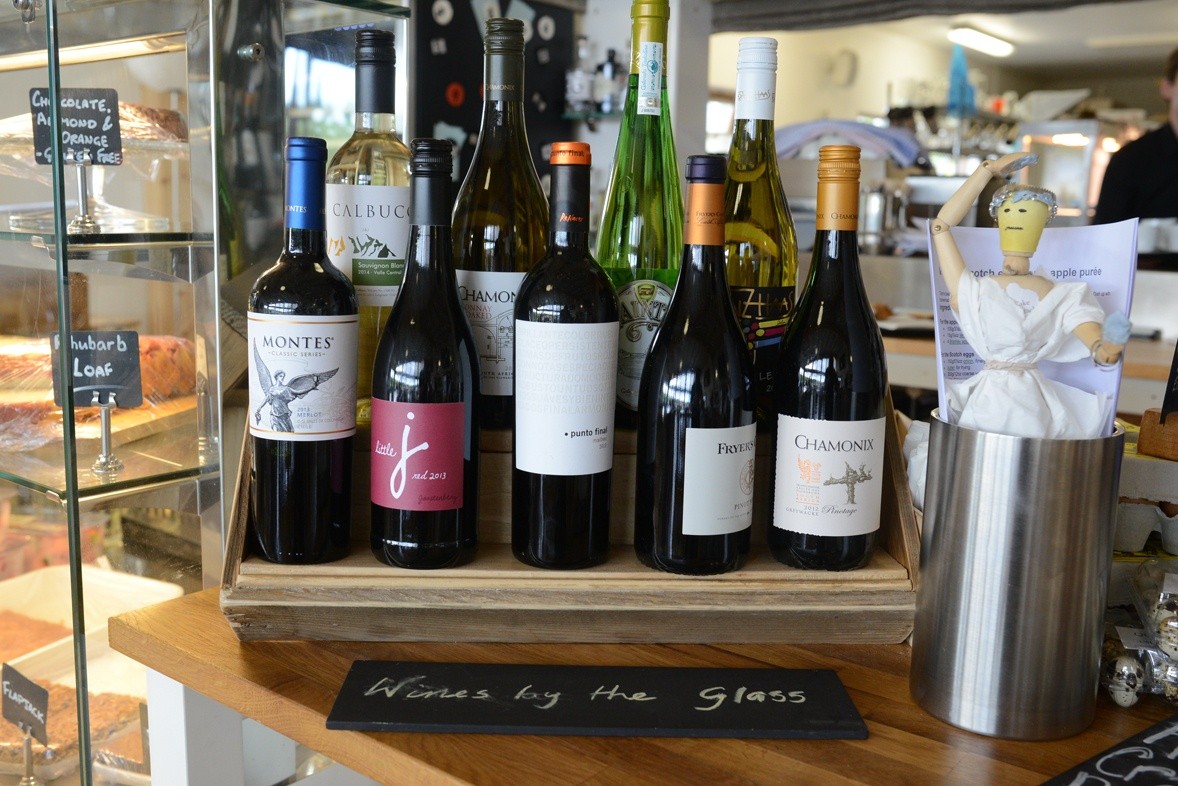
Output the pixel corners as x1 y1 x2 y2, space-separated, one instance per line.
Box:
248 135 360 566
450 19 551 428
759 146 888 570
597 48 623 114
722 36 799 419
559 35 596 116
635 153 755 575
327 30 414 428
513 141 620 570
595 1 683 432
370 138 479 570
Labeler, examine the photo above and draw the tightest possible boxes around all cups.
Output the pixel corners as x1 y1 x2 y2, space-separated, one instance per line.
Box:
909 407 1124 742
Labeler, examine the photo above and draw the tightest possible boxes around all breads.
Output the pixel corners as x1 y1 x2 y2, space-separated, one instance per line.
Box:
0 679 146 763
0 334 197 425
1 98 188 141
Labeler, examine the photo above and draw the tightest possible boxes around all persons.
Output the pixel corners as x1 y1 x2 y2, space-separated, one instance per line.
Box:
932 153 1126 439
1090 48 1178 274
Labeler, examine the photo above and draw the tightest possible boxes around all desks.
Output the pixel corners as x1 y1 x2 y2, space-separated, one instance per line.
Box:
110 584 1178 786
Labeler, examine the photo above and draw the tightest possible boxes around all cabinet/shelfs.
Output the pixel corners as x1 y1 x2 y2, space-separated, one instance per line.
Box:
0 1 420 785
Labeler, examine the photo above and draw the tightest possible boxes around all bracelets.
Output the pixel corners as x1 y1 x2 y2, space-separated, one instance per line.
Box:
1091 340 1123 373
